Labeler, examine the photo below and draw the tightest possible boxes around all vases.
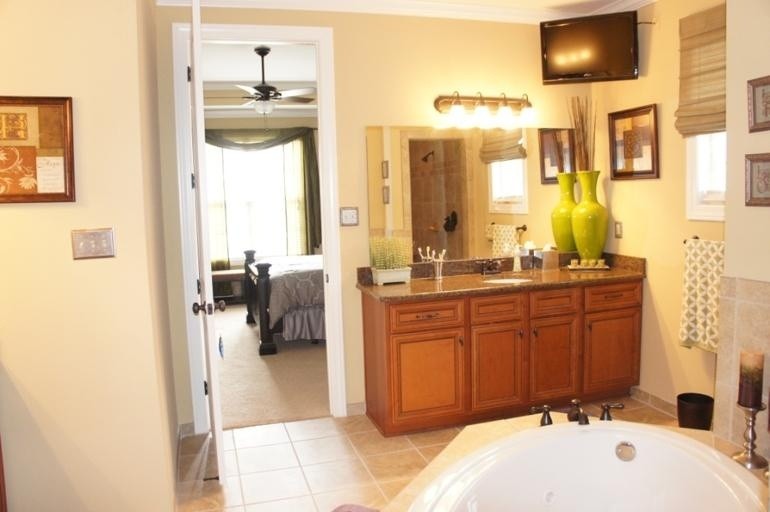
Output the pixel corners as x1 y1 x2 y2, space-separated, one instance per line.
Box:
571 169 608 264
551 173 578 254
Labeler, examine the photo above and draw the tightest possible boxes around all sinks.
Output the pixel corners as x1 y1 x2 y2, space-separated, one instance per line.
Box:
480 278 532 284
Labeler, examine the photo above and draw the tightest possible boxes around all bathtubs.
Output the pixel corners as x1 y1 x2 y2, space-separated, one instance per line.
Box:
407 420 770 512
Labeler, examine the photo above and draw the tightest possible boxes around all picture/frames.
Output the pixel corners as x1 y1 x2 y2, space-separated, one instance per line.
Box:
608 103 659 180
72 228 114 260
538 128 577 185
745 154 770 206
747 75 770 133
341 208 359 226
1 96 76 203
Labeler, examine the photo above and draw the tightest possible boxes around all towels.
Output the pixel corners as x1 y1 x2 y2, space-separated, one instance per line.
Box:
486 224 520 259
678 238 725 354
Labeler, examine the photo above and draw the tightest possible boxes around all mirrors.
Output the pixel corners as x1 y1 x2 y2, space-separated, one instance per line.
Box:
364 125 581 264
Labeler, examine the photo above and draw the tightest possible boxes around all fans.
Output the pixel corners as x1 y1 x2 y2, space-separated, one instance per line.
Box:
234 45 317 135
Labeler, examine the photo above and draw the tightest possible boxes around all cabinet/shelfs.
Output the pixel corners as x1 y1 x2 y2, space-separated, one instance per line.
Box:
363 291 469 438
582 281 644 405
469 286 582 425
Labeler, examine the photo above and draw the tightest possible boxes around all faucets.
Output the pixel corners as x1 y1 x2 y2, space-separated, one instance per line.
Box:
566 398 589 426
488 257 494 270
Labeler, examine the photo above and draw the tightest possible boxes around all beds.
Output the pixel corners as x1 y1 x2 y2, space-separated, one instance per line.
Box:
243 250 326 355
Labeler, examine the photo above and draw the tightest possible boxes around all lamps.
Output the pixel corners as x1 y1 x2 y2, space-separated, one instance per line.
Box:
433 91 535 129
253 100 274 135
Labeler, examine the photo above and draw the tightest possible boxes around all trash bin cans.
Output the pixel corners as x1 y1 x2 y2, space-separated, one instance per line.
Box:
677 392 714 430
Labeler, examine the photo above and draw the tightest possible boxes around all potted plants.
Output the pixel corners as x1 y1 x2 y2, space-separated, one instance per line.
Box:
369 237 412 285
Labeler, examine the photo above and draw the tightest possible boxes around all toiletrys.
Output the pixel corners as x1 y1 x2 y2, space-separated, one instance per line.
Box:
512 246 521 272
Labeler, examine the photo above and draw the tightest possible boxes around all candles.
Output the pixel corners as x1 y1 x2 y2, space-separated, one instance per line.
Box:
738 350 764 408
